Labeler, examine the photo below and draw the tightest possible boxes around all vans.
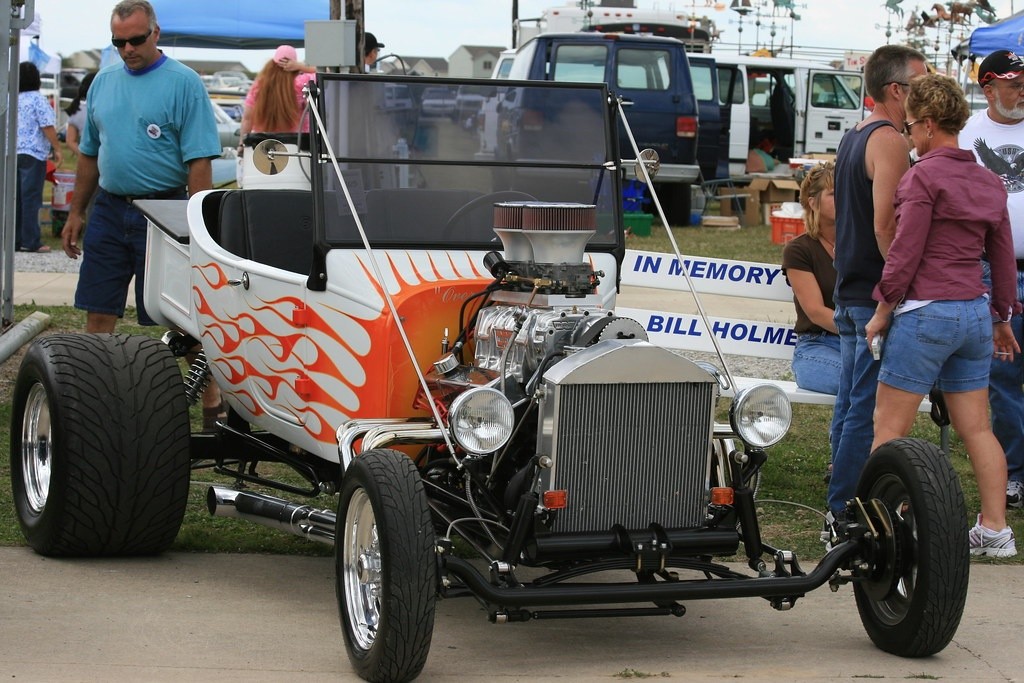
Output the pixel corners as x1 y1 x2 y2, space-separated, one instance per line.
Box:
712 54 874 176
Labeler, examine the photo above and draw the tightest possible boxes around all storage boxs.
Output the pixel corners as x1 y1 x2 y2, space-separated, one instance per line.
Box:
760 202 785 225
720 188 761 226
769 215 805 244
749 177 801 202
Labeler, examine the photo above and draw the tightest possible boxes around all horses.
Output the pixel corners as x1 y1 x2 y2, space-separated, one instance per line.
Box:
906 0 997 32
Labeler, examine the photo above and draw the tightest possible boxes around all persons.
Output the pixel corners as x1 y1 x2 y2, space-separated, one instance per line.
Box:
819 46 930 551
237 45 317 158
909 50 1024 508
6 62 63 253
364 31 385 74
865 73 1021 557
60 0 230 433
67 72 99 232
963 53 980 82
781 162 842 396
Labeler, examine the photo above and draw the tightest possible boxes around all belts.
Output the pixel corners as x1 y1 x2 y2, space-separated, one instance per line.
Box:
97 185 187 205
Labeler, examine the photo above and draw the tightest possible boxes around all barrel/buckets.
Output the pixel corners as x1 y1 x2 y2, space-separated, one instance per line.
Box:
50 173 76 211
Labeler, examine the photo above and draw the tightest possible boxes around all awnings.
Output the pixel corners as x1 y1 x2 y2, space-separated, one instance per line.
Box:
951 9 1024 66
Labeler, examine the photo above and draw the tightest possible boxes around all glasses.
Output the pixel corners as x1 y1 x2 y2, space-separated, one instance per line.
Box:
112 30 152 48
903 119 924 135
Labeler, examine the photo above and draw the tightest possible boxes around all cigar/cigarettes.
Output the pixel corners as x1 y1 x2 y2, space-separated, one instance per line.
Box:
994 352 1012 355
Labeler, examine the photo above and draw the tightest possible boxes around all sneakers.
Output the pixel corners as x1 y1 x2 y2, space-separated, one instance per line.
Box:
1006 480 1024 508
821 510 836 552
968 514 1017 558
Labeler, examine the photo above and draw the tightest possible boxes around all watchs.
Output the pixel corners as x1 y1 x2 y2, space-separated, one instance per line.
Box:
239 143 246 148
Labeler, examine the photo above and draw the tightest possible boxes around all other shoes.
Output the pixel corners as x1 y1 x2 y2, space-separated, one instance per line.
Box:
823 472 831 484
20 245 51 253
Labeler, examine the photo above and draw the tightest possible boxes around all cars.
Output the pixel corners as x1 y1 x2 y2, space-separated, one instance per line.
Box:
496 32 719 226
375 84 412 113
39 77 56 106
474 49 518 163
8 72 970 683
455 84 482 125
421 87 458 118
200 71 255 190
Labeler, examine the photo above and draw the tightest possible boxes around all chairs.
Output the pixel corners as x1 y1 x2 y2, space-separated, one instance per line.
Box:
218 189 338 276
366 189 488 242
769 85 797 147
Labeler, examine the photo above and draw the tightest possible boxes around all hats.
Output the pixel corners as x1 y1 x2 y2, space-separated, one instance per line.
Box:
365 32 384 50
273 45 297 64
978 50 1024 88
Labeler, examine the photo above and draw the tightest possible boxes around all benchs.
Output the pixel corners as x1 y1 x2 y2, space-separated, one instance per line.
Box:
720 373 951 461
